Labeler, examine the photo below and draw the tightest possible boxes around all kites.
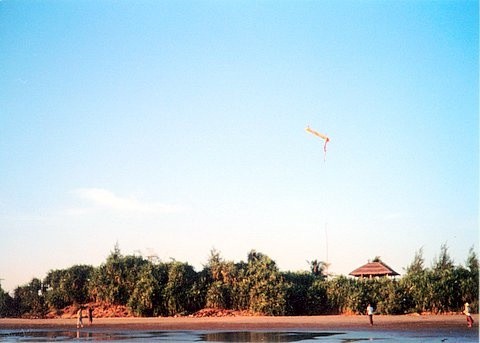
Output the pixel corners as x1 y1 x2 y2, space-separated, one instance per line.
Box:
306 127 330 160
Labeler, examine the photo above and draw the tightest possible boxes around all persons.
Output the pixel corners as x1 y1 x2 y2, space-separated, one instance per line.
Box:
463 302 473 328
77 308 83 329
87 306 94 325
366 304 374 326
